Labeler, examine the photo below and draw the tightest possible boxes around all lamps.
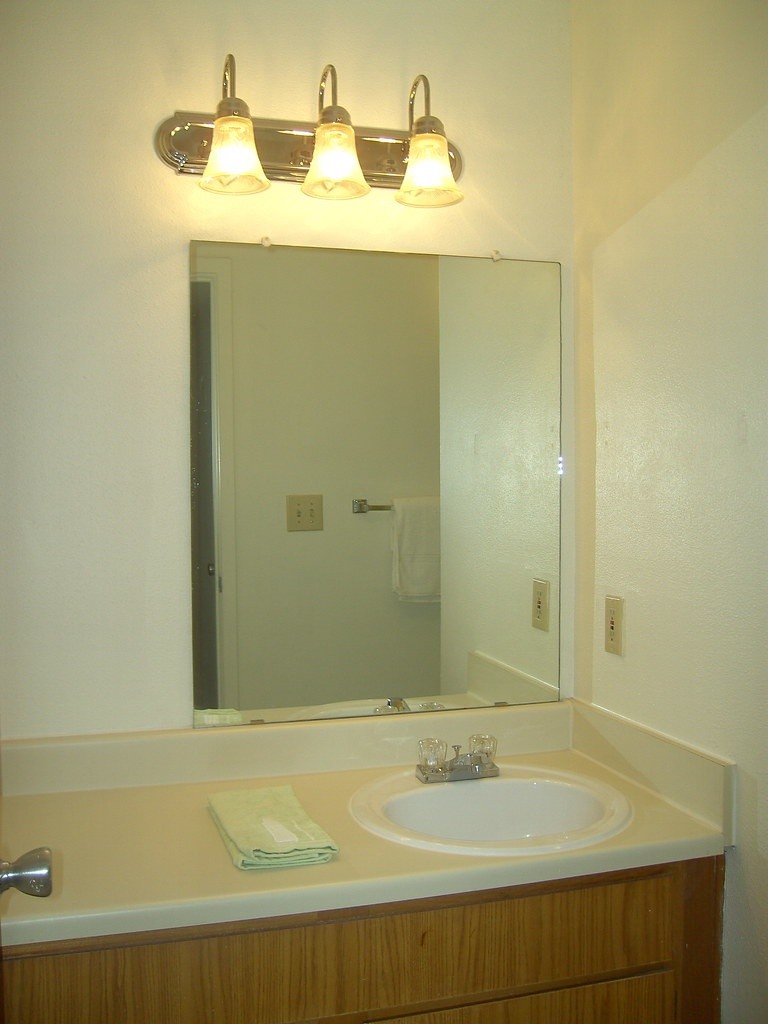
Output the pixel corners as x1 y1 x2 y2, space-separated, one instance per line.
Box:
197 54 271 193
301 64 373 200
395 75 464 208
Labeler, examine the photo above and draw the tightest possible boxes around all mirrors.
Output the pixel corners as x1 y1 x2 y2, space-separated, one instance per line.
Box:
189 239 561 728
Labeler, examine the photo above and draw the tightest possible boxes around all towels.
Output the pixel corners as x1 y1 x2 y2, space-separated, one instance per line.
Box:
389 493 441 605
193 706 242 730
208 780 339 869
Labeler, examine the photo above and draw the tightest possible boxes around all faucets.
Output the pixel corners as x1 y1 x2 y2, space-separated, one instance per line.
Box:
449 753 473 772
387 695 411 712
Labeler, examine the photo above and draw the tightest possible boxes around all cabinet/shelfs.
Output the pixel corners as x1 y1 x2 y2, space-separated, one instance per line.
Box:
361 968 677 1024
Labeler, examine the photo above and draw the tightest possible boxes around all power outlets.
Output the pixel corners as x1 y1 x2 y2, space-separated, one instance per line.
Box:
530 578 549 632
604 595 623 656
285 494 323 531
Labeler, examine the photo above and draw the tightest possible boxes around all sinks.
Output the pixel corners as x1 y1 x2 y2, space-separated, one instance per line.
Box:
348 759 637 856
290 697 460 722
